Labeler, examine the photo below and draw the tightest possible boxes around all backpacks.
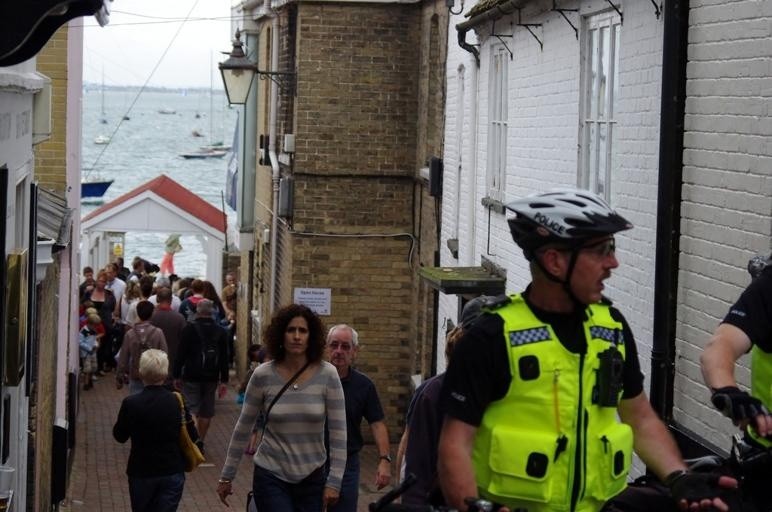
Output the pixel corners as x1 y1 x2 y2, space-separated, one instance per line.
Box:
130 325 157 380
186 319 221 376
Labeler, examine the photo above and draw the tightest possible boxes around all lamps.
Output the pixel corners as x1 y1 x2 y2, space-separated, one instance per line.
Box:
216 26 297 106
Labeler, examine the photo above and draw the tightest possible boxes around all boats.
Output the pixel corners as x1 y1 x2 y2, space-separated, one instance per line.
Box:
82 165 116 204
92 134 111 145
175 148 231 159
158 108 178 117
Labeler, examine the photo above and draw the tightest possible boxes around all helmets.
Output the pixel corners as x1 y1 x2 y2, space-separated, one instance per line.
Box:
504 188 633 259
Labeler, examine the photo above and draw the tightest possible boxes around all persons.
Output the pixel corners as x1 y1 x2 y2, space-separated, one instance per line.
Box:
111 348 205 511
435 188 742 510
213 304 348 511
391 293 511 511
78 255 268 454
326 324 393 511
699 253 771 453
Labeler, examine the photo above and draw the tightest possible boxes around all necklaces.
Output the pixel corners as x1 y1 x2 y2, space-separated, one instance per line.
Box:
287 365 311 390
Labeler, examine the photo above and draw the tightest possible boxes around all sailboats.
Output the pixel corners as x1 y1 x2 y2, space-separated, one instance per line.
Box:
199 52 231 151
97 64 110 126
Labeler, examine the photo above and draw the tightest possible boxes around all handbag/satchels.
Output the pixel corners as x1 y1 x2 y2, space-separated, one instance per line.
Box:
180 422 207 472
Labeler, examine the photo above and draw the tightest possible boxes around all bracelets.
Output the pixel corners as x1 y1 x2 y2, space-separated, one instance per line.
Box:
663 469 688 485
379 455 392 462
217 478 229 483
